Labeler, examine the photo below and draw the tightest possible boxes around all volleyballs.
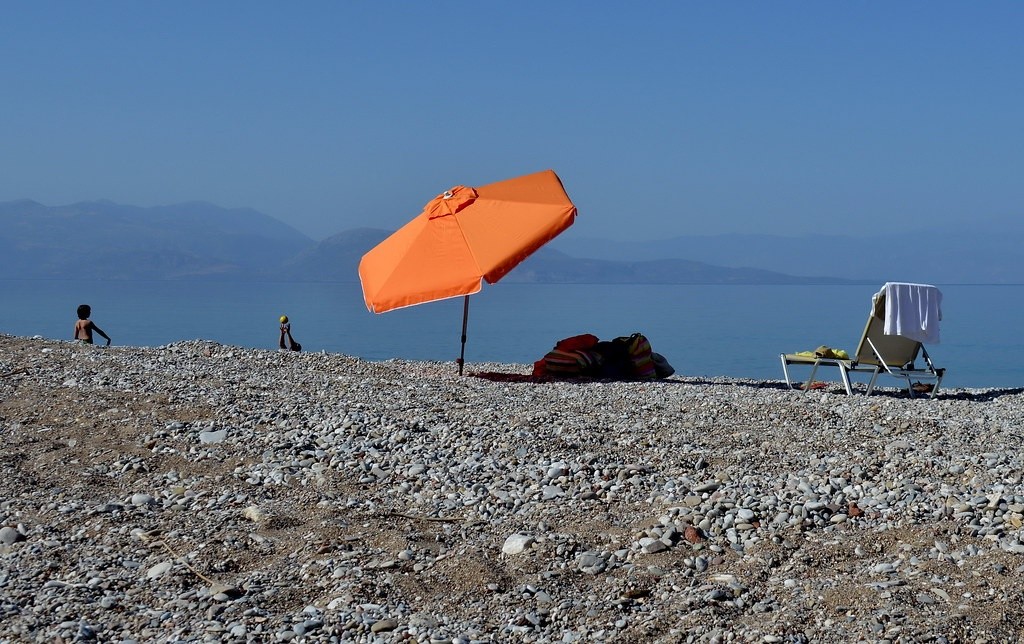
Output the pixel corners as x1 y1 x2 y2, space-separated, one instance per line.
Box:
279 315 288 324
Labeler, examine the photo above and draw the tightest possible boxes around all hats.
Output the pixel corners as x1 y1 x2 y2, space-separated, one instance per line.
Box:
813 345 835 358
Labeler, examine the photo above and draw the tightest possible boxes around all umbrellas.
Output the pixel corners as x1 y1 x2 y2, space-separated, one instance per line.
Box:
358 170 577 376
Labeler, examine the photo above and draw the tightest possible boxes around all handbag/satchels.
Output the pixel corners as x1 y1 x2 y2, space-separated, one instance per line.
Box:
612 332 656 382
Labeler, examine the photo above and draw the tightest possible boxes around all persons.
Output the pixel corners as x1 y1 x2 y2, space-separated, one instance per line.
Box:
74 305 111 346
279 323 302 352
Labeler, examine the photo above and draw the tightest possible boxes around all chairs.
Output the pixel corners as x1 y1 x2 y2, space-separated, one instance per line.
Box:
780 281 947 398
